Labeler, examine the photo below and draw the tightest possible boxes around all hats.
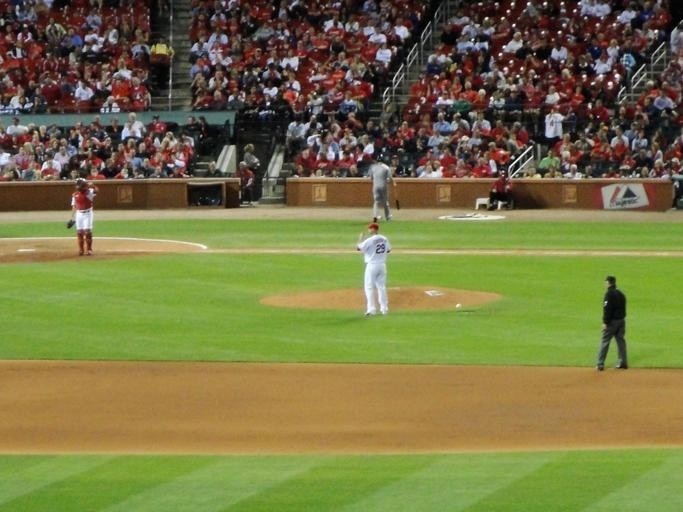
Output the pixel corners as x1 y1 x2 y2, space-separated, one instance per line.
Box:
368 223 380 231
604 275 617 285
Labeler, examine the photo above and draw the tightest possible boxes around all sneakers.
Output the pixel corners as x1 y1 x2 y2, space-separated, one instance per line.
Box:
373 214 393 223
616 365 629 370
597 365 604 371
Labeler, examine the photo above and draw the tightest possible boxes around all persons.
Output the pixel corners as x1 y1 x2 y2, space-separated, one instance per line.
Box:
355 224 391 318
667 156 683 213
594 275 629 371
65 177 98 257
366 153 395 223
486 170 511 209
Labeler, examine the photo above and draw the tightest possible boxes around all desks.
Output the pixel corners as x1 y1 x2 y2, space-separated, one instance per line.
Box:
0 176 243 210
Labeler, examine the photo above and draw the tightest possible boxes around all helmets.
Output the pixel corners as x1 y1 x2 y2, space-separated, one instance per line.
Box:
376 153 384 162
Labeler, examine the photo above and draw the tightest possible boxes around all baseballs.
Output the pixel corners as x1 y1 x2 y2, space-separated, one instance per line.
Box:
455 304 463 310
